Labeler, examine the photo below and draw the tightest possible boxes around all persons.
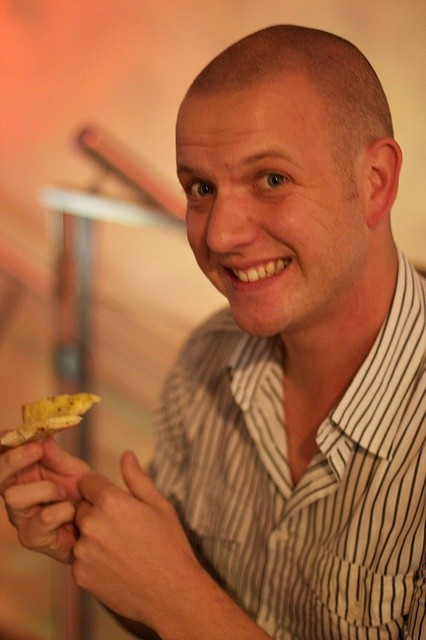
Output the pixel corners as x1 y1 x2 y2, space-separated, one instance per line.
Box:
0 26 426 640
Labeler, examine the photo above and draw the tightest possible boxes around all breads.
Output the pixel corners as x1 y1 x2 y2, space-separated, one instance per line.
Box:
0 393 101 447
